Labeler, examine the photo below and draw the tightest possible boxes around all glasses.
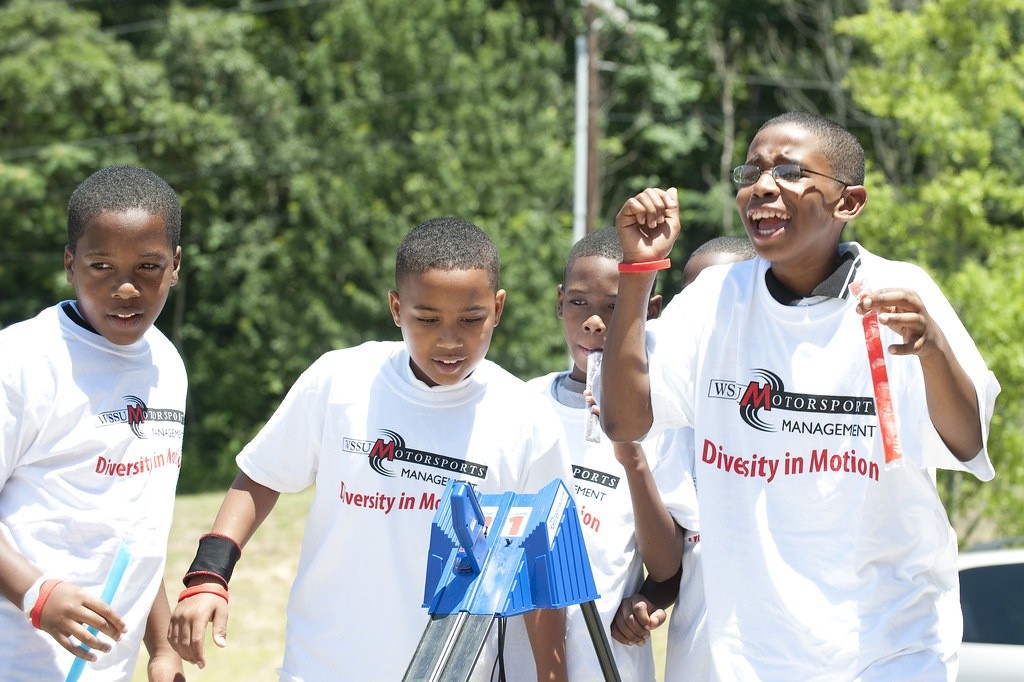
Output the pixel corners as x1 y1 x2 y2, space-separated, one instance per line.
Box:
728 164 852 187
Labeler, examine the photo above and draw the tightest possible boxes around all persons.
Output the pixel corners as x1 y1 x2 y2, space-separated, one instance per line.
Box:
527 227 701 682
600 111 1003 682
0 165 188 682
166 218 574 681
609 235 758 646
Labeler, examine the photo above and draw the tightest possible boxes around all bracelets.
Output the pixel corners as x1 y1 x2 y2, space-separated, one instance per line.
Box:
178 586 229 602
24 577 51 623
618 259 672 273
182 534 241 585
32 579 64 629
